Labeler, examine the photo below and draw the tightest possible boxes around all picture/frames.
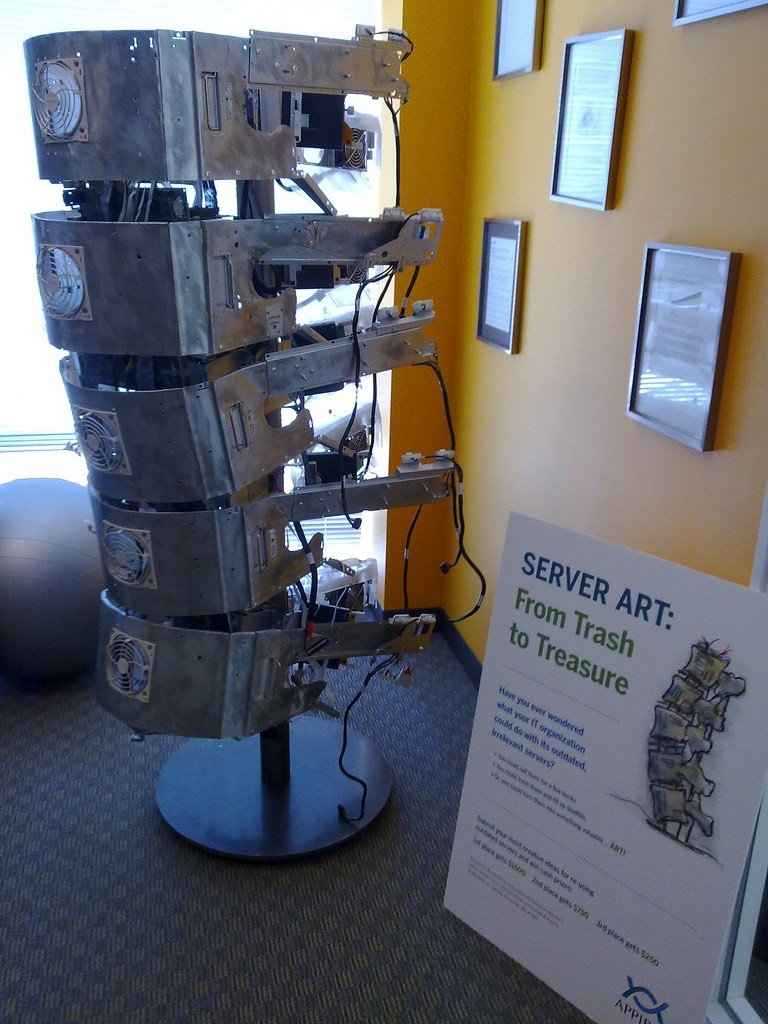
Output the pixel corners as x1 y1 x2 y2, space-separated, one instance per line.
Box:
493 0 546 81
476 217 528 355
547 28 634 211
624 240 743 452
673 0 768 27
